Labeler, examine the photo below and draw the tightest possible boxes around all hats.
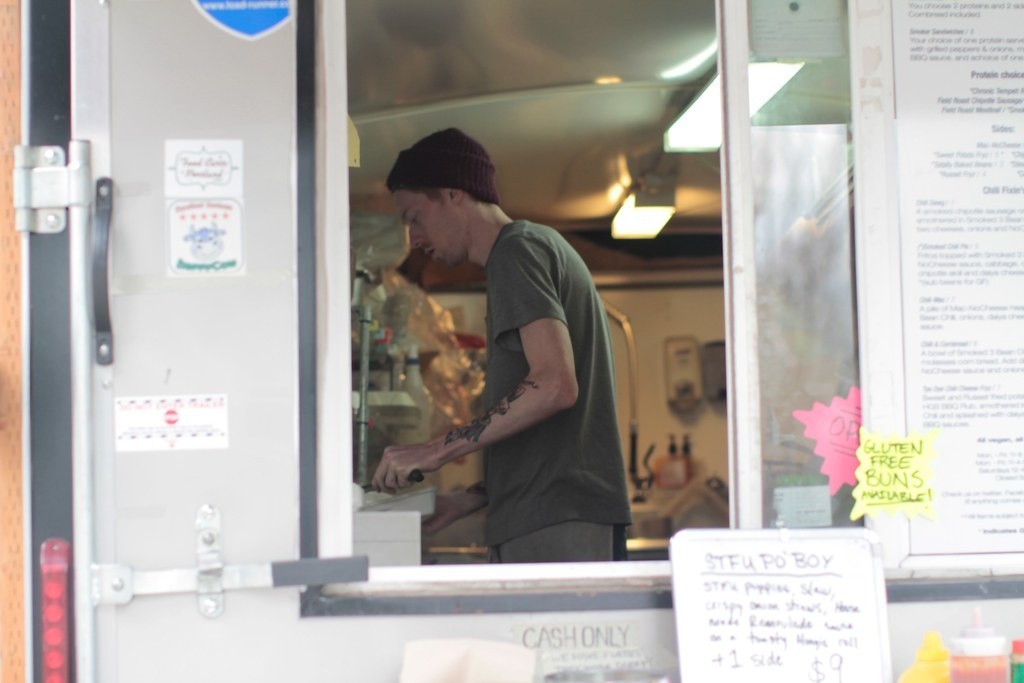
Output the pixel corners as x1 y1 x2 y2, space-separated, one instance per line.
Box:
386 128 499 204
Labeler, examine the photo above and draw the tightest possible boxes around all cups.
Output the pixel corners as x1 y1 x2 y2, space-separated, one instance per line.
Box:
950 638 1010 683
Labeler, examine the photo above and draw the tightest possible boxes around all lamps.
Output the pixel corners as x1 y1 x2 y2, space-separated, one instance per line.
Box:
610 172 676 241
663 49 805 157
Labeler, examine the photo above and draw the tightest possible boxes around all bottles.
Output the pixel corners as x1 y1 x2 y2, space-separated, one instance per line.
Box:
681 437 699 487
1012 640 1024 683
390 344 432 485
896 633 950 683
654 435 686 489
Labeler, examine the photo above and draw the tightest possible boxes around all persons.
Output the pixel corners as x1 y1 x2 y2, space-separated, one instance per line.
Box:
371 128 632 564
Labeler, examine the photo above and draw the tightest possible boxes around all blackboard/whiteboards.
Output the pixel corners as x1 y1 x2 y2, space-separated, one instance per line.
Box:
666 527 893 683
